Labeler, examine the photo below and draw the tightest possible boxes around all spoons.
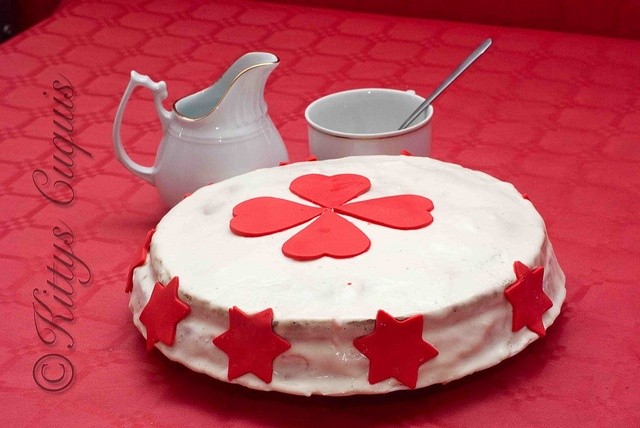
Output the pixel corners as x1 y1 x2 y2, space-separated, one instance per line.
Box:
389 39 497 145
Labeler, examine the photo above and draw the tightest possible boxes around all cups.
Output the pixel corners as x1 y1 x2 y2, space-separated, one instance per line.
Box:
112 49 286 206
304 87 439 158
